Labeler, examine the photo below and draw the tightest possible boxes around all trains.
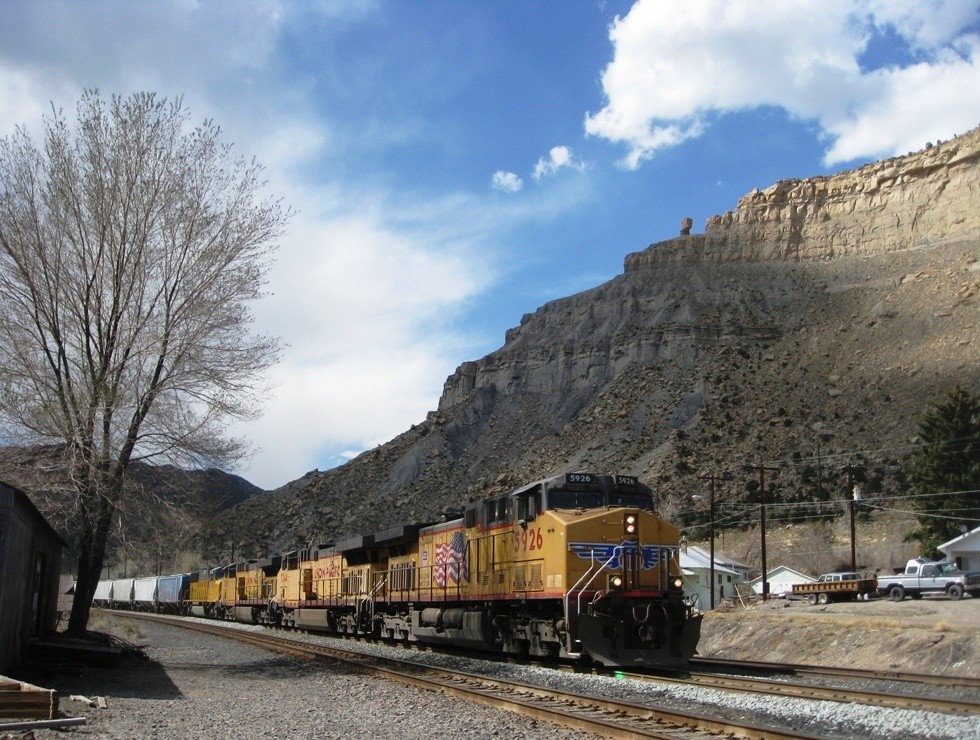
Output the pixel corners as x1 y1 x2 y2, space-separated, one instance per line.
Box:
74 472 703 675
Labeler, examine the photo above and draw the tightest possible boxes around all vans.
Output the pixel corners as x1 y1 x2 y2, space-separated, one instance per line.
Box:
807 572 862 583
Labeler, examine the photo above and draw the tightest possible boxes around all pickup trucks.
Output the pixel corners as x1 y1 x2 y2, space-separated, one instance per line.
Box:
874 558 980 602
